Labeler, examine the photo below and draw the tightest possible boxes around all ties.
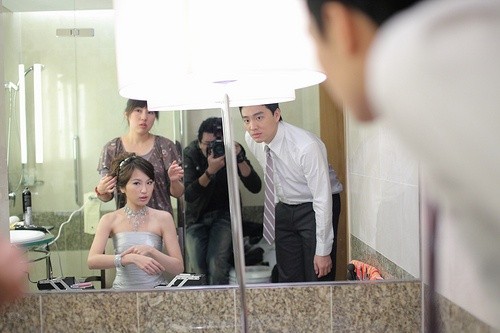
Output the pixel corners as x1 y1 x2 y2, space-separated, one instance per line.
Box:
262 146 276 245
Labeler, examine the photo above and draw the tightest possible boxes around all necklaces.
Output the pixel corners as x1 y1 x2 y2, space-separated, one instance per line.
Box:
124 204 151 231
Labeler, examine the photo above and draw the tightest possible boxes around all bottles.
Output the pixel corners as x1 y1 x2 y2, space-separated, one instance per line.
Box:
22 187 32 229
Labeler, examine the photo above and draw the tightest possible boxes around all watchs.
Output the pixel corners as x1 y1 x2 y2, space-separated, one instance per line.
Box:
205 170 216 180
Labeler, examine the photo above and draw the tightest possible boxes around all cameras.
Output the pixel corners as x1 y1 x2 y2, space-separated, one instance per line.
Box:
206 126 225 159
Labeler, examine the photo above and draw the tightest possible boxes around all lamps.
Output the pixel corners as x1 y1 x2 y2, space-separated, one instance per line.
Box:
112 1 324 333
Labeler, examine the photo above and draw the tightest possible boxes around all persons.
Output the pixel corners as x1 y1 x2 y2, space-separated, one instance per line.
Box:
87 153 185 290
95 99 186 223
239 104 343 282
182 117 263 286
0 242 33 305
304 0 500 333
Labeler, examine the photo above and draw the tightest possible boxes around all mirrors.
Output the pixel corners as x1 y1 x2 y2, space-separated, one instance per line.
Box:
1 1 423 294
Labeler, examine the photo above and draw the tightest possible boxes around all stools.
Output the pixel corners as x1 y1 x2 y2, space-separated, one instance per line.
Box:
10 227 58 290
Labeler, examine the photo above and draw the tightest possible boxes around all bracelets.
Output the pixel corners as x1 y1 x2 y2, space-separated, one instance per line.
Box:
114 254 124 269
96 187 107 196
